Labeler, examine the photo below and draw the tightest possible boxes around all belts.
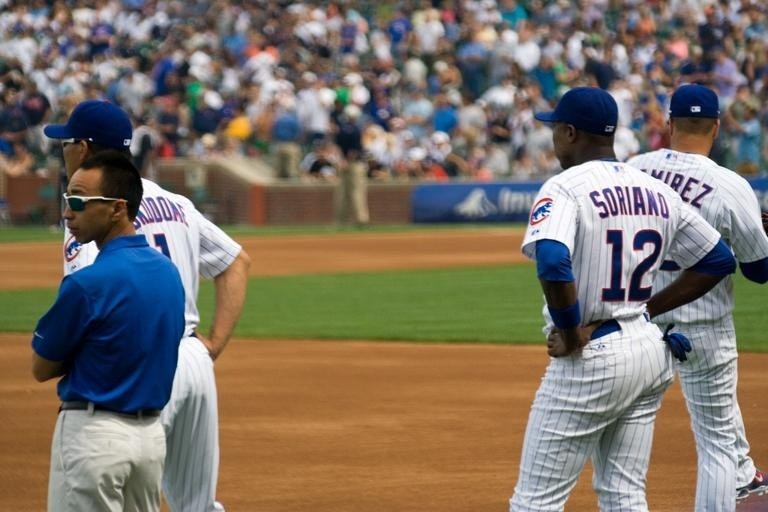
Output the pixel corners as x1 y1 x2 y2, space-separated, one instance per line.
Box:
59 400 161 417
589 312 649 340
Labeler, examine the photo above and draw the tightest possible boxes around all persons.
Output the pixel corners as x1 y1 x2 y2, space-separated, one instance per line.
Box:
0 0 768 228
31 149 186 512
626 84 768 512
509 87 738 512
44 101 251 512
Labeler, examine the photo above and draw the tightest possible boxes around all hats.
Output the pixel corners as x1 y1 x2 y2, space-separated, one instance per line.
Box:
44 101 131 151
671 85 720 120
535 86 617 136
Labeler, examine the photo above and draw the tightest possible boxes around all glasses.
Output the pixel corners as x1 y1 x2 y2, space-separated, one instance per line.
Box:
61 138 93 147
61 193 126 211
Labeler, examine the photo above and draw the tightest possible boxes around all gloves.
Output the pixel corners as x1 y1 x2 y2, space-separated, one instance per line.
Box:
664 325 690 362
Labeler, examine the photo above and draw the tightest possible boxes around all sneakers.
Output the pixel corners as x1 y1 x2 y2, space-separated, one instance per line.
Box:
737 467 768 501
